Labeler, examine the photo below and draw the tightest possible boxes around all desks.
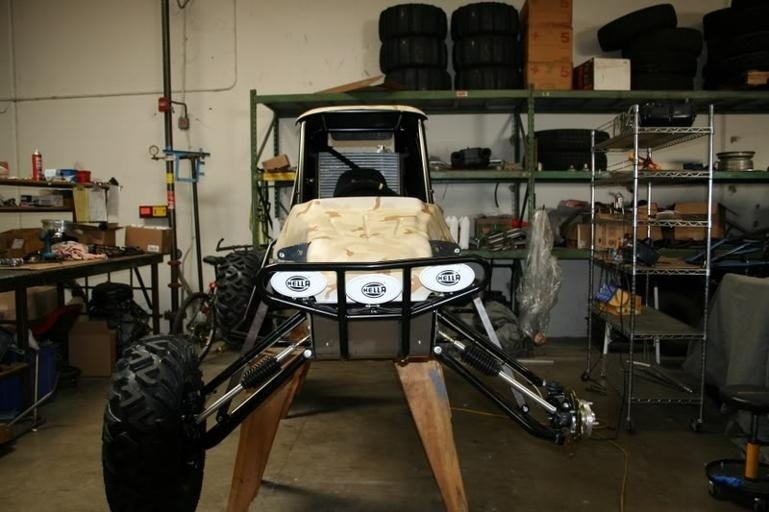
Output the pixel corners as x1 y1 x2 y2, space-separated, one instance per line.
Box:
2 251 165 430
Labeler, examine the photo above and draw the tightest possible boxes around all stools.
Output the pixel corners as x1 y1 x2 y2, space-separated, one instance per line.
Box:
703 382 769 512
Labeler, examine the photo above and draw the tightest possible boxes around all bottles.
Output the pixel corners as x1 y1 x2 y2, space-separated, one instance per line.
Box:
33 147 43 181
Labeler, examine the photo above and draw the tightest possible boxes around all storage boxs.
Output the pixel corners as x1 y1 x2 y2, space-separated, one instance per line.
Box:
62 318 117 379
518 1 633 92
78 218 173 256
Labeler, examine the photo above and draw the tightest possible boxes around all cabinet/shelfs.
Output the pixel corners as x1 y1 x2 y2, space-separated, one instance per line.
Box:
242 83 769 435
